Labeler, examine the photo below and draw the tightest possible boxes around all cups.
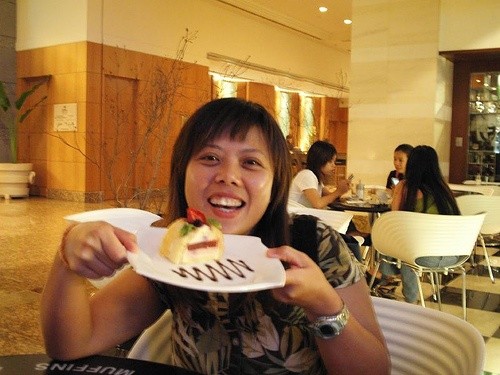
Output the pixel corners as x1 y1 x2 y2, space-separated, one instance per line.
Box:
376 190 386 204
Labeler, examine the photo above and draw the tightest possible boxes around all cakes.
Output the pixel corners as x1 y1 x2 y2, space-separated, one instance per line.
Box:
160 207 223 264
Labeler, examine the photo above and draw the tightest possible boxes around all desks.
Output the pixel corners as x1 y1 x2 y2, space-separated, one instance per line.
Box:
329 194 392 224
0 354 203 375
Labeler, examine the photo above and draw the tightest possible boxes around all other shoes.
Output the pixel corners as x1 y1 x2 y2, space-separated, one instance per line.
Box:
366 272 382 290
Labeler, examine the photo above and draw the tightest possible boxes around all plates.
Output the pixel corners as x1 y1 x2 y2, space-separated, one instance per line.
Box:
125 227 287 293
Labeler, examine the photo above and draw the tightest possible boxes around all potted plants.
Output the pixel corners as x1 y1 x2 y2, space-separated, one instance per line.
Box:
0 79 47 201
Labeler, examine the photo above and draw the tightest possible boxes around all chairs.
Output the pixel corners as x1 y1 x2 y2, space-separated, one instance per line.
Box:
127 294 487 375
447 179 500 283
367 210 489 319
287 209 353 235
286 199 306 214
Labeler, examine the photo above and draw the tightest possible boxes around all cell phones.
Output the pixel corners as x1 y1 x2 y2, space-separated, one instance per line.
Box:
347 174 354 181
391 178 399 186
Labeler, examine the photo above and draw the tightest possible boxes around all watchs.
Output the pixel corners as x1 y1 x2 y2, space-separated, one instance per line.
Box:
307 304 349 340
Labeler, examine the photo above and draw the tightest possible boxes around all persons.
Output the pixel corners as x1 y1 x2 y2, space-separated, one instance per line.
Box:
386 144 414 275
42 98 392 375
287 141 362 264
378 145 463 304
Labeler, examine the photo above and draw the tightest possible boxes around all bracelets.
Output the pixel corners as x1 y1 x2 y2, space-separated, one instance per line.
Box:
60 222 77 265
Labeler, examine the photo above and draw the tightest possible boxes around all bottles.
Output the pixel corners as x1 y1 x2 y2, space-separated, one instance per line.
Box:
356 178 366 202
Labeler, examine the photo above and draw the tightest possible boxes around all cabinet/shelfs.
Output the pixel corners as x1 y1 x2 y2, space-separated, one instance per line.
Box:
438 47 500 196
